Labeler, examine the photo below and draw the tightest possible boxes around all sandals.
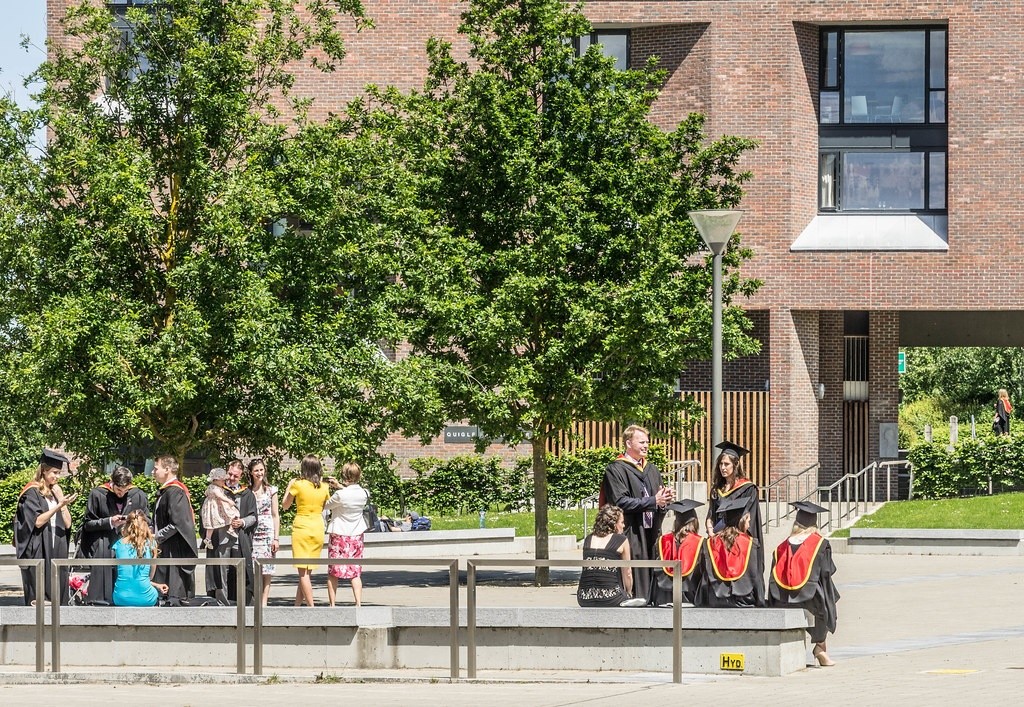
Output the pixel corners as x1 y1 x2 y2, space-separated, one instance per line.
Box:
226 529 239 539
203 538 214 549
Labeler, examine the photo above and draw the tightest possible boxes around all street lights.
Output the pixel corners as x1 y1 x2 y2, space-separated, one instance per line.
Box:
688 210 743 494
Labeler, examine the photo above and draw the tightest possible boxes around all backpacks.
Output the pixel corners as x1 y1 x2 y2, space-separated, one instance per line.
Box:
409 517 431 530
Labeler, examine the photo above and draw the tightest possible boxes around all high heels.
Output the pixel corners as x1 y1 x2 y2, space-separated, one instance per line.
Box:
813 645 835 668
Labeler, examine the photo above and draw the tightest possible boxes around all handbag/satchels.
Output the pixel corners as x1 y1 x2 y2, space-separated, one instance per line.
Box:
363 489 381 533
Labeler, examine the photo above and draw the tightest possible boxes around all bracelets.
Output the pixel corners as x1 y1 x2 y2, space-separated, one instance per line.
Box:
61 508 66 512
274 538 279 541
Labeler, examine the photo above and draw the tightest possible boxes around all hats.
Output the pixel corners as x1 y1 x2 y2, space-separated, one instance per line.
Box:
407 510 419 520
40 449 74 476
715 441 750 460
665 499 706 523
210 468 229 480
788 502 830 529
716 497 751 517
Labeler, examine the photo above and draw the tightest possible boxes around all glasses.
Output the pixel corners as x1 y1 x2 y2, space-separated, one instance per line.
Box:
250 458 263 464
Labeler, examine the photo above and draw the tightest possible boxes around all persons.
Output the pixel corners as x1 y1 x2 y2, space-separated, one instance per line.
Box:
282 453 330 607
199 458 258 606
769 501 840 666
112 510 169 608
702 497 765 610
202 467 240 549
993 388 1011 435
602 425 676 604
14 449 78 605
380 511 419 531
325 460 370 608
647 499 708 608
81 466 151 607
577 505 633 607
248 459 280 607
151 455 198 598
705 440 762 537
